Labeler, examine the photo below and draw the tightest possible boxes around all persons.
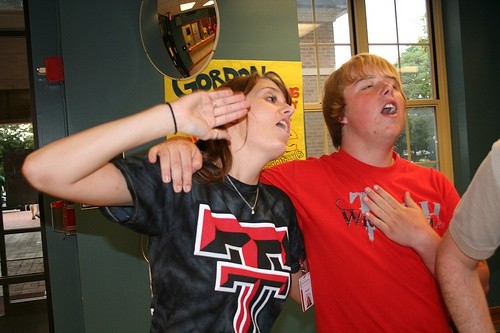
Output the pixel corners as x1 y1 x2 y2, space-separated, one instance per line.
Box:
21 70 315 332
148 54 490 333
436 120 500 333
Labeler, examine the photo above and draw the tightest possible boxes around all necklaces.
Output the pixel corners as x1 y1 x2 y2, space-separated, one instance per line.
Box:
214 163 260 215
